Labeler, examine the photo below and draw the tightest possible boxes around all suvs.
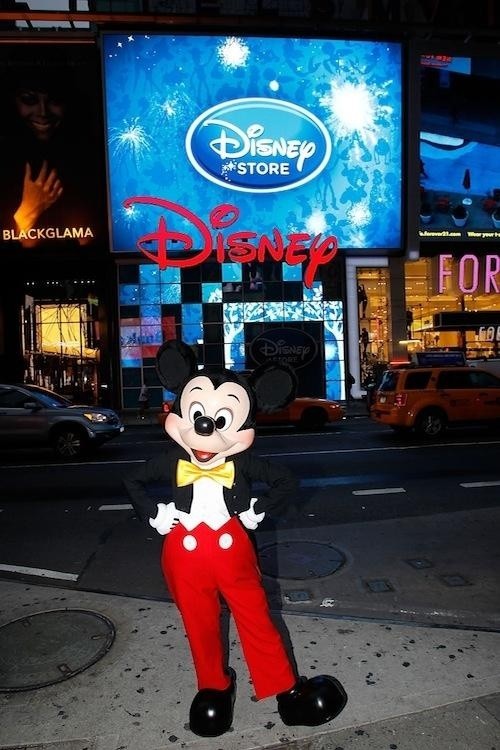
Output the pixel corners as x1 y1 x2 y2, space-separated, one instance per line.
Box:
368 364 500 440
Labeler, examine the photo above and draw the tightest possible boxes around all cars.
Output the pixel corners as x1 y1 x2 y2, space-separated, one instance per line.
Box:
1 382 129 462
155 369 345 433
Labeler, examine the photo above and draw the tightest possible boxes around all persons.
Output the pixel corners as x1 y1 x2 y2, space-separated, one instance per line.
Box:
361 328 369 352
0 65 97 242
137 379 149 420
343 372 355 403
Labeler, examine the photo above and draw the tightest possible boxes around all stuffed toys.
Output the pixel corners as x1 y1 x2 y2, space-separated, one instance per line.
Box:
120 339 348 737
